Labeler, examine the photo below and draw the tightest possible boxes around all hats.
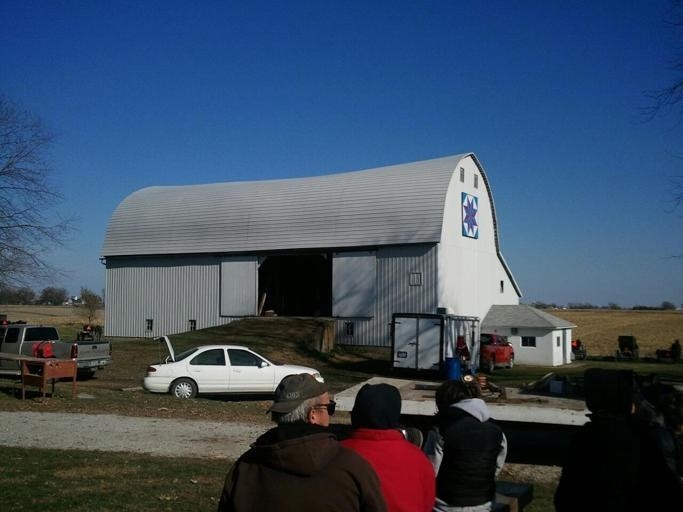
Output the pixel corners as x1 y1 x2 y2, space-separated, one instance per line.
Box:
264 374 326 415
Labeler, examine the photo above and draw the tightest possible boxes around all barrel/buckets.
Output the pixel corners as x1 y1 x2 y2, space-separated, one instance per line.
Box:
445 356 461 379
478 376 486 387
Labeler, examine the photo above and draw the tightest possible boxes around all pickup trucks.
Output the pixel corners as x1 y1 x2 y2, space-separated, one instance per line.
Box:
0 322 112 381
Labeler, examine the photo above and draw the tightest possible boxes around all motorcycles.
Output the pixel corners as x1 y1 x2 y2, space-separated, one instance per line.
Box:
571 339 586 360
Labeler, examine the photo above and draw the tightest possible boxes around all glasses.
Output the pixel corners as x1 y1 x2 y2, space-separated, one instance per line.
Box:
307 399 336 417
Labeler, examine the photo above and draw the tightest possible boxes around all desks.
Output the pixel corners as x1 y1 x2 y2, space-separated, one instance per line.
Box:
18 358 78 402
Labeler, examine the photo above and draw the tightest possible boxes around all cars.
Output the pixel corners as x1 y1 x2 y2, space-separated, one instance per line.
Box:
143 335 325 398
480 333 515 372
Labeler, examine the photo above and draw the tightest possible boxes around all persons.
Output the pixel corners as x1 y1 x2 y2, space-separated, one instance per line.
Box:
217 373 387 512
553 368 683 512
423 380 508 512
338 384 436 512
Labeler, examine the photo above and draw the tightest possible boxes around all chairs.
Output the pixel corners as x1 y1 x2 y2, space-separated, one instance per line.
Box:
216 356 224 363
